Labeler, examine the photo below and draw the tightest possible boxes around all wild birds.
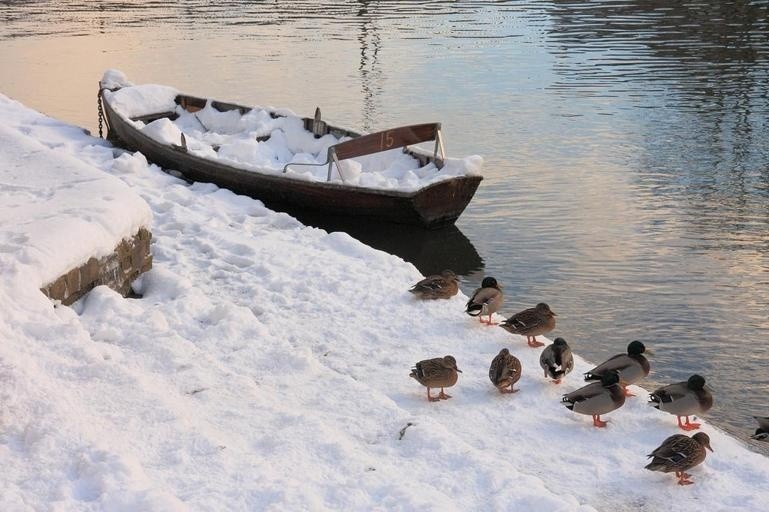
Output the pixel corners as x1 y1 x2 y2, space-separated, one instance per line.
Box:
497 302 558 347
488 347 522 394
407 268 462 300
748 415 768 441
646 374 716 431
464 275 504 325
409 355 463 402
560 340 655 427
643 432 714 486
539 338 574 385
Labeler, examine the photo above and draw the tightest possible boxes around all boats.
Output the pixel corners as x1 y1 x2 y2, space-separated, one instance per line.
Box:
98 69 486 234
